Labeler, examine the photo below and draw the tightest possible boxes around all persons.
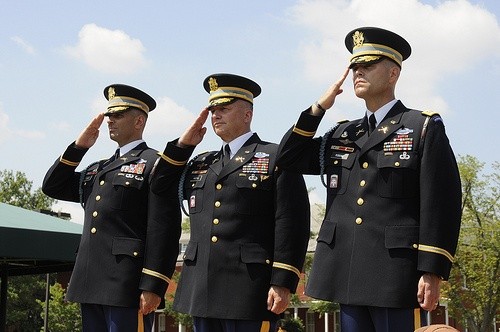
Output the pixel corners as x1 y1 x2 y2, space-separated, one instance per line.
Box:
275 27 462 332
42 84 182 332
150 73 310 332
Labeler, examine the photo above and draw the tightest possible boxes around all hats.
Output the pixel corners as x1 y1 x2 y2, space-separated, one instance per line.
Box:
345 27 412 70
104 84 156 116
203 73 261 110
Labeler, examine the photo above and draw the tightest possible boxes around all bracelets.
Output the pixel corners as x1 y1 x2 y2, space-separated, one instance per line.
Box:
315 101 326 112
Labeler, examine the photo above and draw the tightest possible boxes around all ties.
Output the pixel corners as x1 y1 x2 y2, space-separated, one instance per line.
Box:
115 149 120 160
369 114 376 137
223 144 230 168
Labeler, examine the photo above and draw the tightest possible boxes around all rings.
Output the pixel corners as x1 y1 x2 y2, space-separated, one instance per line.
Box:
153 307 157 311
434 302 438 305
